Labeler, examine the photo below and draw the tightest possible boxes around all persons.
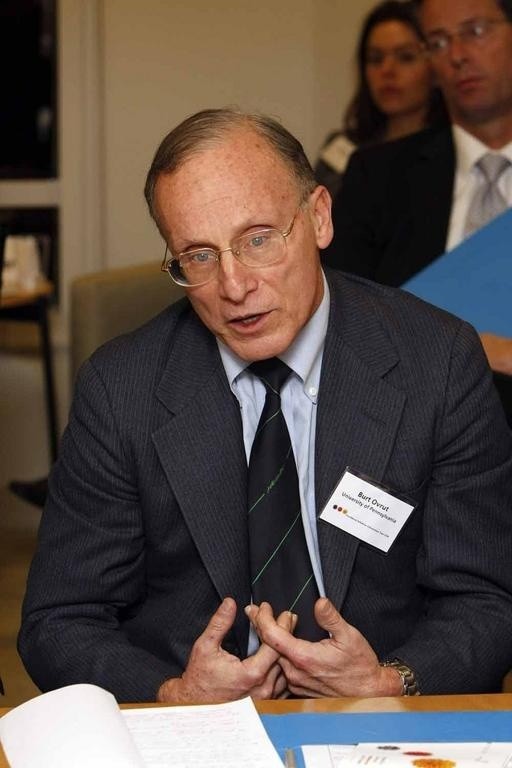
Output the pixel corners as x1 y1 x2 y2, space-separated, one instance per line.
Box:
17 104 512 705
313 1 455 203
322 1 511 287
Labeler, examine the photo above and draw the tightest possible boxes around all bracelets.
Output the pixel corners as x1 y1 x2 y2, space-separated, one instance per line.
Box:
380 658 421 696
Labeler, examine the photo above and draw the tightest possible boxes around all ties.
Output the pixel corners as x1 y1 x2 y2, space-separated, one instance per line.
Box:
244 356 336 644
464 154 509 237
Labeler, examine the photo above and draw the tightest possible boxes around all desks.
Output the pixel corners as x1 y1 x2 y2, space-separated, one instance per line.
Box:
0 688 509 768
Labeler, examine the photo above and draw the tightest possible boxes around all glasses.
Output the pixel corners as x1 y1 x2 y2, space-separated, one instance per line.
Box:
421 16 505 56
159 196 308 288
